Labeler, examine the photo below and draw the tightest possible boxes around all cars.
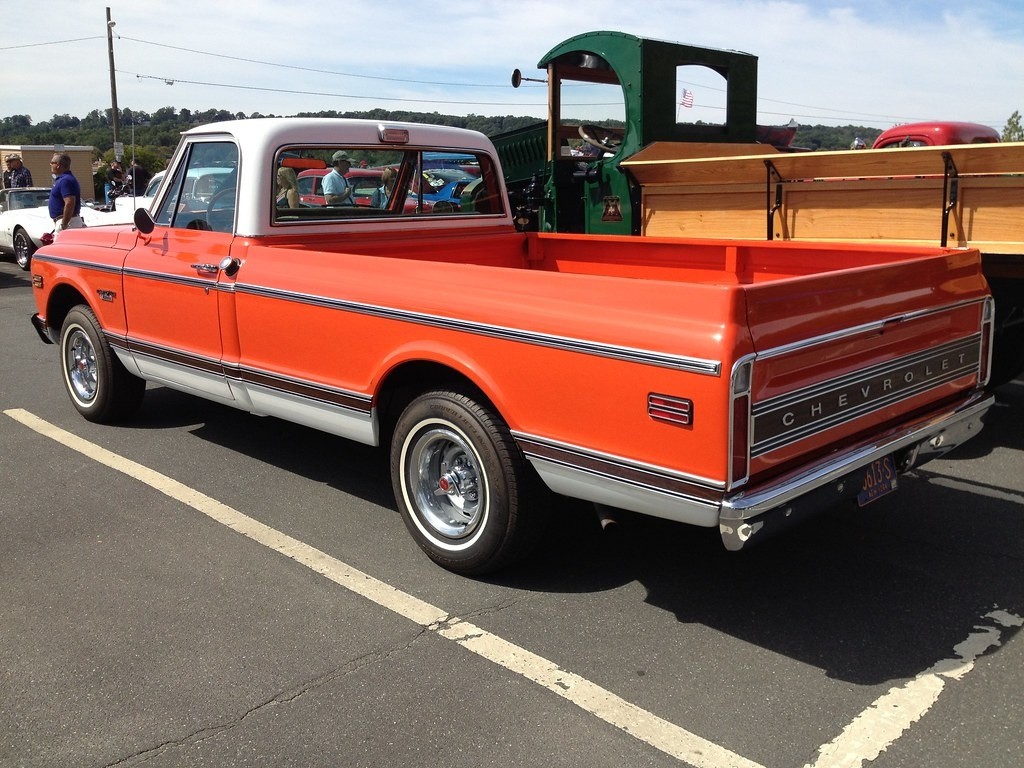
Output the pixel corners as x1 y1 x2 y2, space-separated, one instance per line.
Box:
0 151 490 283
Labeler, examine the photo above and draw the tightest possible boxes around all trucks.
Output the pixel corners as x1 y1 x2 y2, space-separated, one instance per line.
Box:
491 32 1024 398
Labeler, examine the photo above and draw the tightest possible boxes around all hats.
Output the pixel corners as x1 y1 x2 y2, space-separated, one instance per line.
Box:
7 153 20 160
332 150 356 163
111 160 122 164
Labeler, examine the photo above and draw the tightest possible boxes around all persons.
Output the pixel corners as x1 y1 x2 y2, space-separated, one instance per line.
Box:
106 160 122 188
48 153 83 235
127 158 151 196
370 166 398 209
276 167 300 209
359 160 368 169
6 153 33 188
577 140 599 157
3 155 13 189
321 150 357 205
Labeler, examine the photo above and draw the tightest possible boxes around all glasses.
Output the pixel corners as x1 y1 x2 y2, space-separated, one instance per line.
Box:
50 161 63 166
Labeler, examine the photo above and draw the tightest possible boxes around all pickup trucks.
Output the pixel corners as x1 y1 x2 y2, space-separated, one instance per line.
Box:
29 117 994 577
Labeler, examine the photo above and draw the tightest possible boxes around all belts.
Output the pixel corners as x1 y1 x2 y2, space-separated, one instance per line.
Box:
54 214 79 223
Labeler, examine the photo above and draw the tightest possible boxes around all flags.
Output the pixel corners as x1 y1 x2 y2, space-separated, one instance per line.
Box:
681 89 693 108
